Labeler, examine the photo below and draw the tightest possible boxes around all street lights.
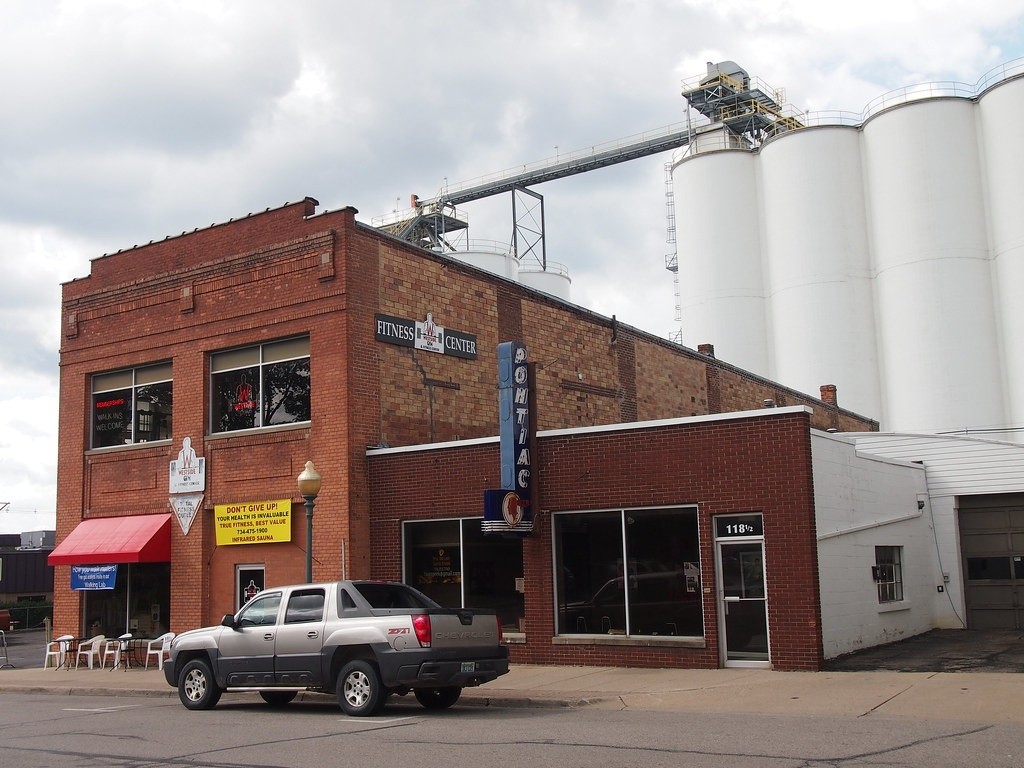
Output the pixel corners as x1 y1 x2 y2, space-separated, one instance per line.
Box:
298 460 321 583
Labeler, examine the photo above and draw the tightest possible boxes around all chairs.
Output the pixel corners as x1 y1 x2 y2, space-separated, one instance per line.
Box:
44 635 74 669
145 632 175 670
75 635 105 669
102 633 132 669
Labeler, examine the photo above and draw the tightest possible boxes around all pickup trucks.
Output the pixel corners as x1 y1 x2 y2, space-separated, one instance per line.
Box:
163 580 510 717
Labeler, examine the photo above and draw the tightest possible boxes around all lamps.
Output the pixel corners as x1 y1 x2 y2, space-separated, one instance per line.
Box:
297 461 322 495
